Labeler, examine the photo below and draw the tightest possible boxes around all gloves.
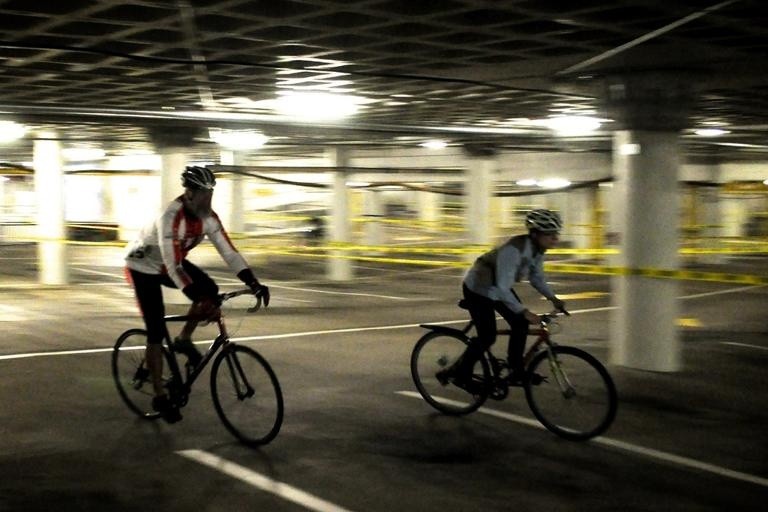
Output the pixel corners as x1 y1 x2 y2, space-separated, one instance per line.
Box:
236 269 269 307
182 283 213 318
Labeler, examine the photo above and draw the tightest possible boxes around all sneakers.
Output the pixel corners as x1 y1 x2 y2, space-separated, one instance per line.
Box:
173 337 202 368
153 395 182 424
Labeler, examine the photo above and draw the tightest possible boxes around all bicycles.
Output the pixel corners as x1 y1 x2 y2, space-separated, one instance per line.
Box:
408 298 620 442
110 289 284 446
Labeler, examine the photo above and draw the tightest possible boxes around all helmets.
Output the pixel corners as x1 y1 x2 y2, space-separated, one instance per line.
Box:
181 167 216 192
525 209 565 232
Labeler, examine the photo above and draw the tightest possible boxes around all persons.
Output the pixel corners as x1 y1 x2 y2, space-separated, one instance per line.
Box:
452 208 566 394
124 165 268 424
